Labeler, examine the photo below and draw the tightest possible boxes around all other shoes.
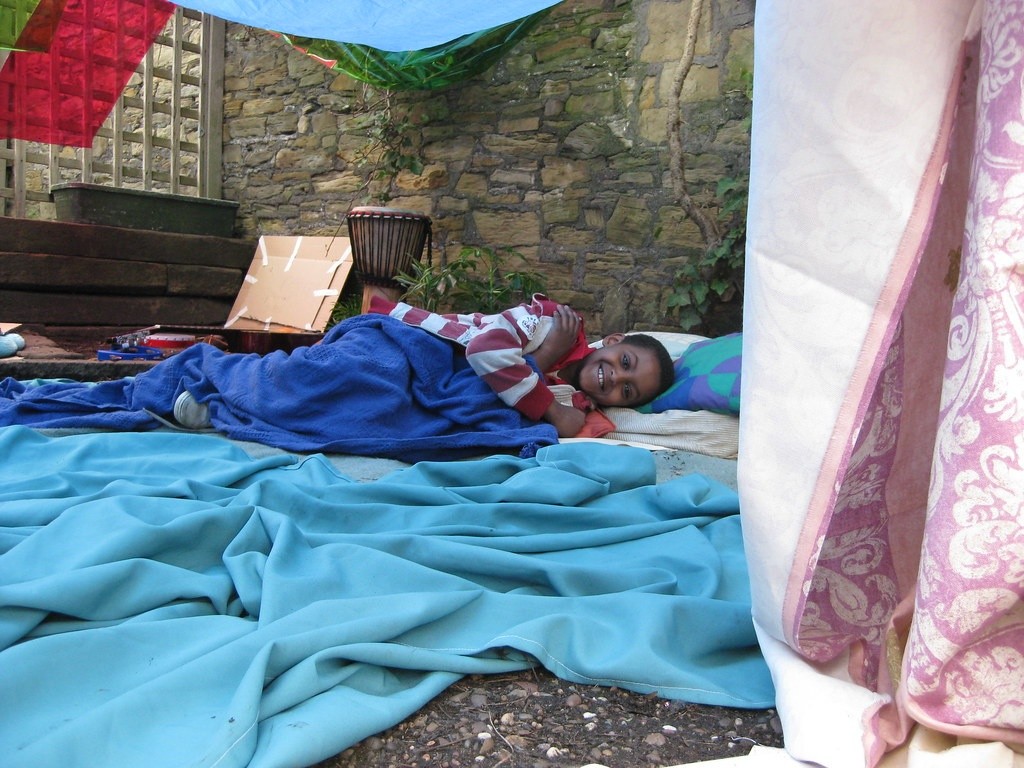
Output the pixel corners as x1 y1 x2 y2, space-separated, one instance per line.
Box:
174 391 210 428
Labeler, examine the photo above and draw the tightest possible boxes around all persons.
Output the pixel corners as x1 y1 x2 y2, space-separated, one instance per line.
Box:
173 293 675 438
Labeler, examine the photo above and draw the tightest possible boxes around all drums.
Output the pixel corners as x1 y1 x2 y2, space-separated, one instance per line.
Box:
140 333 196 355
345 204 432 315
